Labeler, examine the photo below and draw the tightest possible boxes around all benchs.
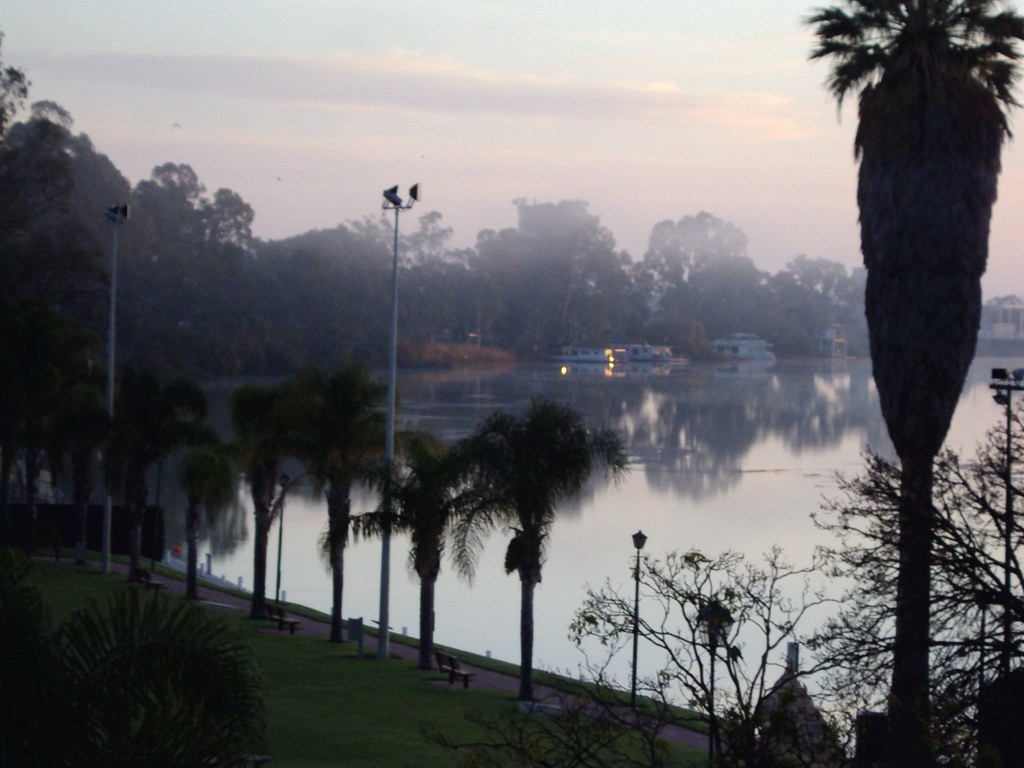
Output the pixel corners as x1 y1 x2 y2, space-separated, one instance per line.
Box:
136 567 164 592
435 650 477 689
264 600 301 635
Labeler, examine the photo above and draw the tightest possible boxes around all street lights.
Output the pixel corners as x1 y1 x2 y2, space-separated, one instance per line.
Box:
628 527 648 711
988 363 1024 731
95 203 132 576
374 181 423 661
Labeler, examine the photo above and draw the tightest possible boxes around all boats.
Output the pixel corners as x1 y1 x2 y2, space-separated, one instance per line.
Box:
544 344 628 366
711 332 775 361
622 344 672 362
